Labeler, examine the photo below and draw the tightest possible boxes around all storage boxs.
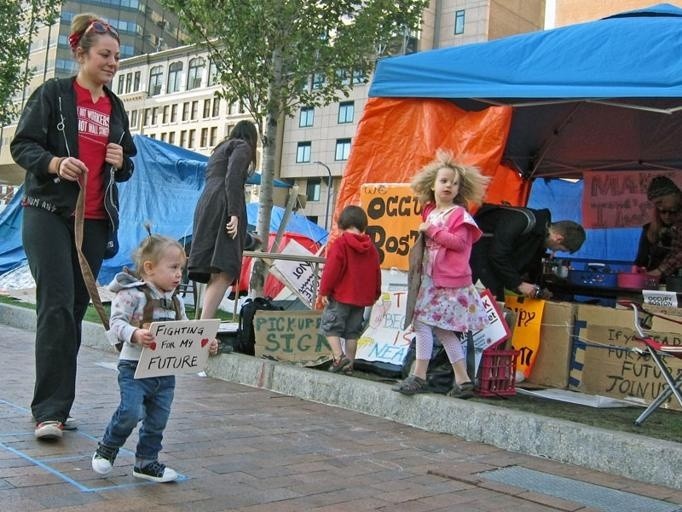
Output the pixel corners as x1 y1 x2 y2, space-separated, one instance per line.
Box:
528 300 682 411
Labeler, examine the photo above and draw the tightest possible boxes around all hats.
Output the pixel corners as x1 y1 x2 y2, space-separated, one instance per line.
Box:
647 175 678 200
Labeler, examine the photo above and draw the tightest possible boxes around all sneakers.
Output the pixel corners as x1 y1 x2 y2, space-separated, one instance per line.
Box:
446 381 475 399
62 414 77 430
35 419 63 441
391 374 431 395
133 460 178 482
92 441 119 475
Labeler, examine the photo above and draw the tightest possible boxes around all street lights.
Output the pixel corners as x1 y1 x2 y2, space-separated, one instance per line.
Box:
312 160 332 231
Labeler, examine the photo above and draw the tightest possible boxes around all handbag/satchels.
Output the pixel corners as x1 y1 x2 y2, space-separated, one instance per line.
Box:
401 331 475 393
474 202 536 239
233 296 282 355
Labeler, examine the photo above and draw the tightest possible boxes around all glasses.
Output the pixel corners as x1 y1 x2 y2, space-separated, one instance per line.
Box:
83 20 119 37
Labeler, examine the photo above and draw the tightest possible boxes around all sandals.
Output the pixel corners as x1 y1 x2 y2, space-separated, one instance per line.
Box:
342 364 354 376
327 351 350 372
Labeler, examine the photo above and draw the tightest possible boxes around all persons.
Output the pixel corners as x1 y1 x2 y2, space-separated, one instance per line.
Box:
451 203 585 341
9 14 137 439
315 206 381 375
187 120 264 320
634 207 672 291
647 177 682 292
391 147 491 399
92 221 219 482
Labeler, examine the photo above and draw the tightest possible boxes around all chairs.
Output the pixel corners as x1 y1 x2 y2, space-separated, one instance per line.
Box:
616 296 682 424
